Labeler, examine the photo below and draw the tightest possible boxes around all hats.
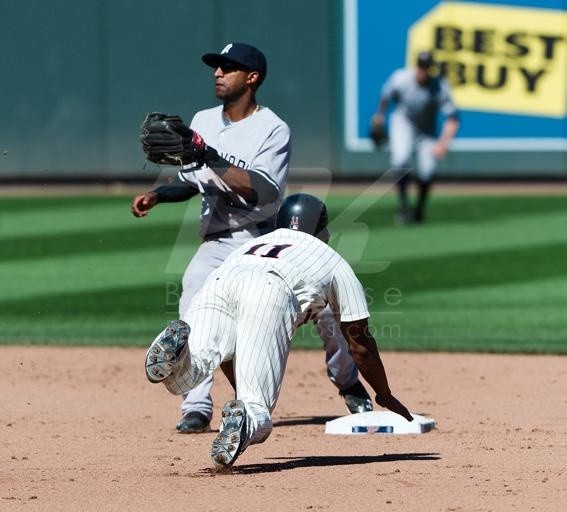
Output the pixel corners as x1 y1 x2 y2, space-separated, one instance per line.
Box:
202 41 267 83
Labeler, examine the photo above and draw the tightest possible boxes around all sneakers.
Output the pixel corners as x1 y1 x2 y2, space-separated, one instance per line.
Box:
210 399 247 468
176 411 213 433
396 208 424 225
145 319 192 383
339 379 373 415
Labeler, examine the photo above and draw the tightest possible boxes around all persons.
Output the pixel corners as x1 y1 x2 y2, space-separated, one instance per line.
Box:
370 53 460 224
128 41 291 435
142 194 414 471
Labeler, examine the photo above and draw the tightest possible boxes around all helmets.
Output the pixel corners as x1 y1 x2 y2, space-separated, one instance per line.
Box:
416 52 434 69
273 193 331 244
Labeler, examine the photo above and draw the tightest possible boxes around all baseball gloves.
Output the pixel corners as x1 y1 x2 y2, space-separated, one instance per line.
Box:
140 111 217 173
369 123 389 146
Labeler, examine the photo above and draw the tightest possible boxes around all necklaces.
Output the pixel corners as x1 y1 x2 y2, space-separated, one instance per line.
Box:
251 105 259 115
